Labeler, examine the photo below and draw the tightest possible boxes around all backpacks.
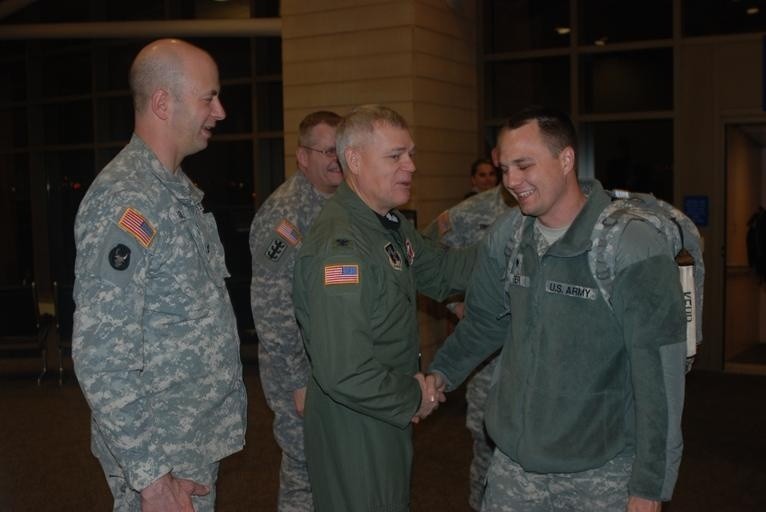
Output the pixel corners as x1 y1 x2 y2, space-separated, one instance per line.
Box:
590 188 704 376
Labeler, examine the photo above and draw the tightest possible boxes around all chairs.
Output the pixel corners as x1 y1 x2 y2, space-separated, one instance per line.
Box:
0 282 75 386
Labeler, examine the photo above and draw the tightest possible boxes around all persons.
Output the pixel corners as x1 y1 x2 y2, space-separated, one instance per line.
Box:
409 106 688 512
68 38 250 512
291 102 484 511
246 110 347 510
465 156 499 195
413 105 524 512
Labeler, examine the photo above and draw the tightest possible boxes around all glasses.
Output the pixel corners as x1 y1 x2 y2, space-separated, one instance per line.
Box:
300 144 337 158
